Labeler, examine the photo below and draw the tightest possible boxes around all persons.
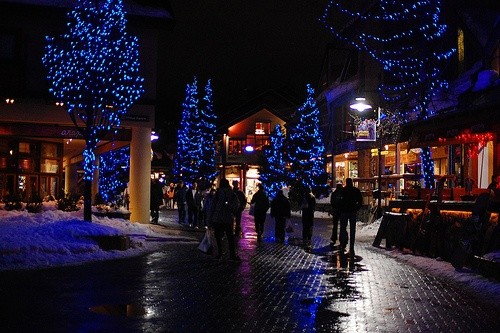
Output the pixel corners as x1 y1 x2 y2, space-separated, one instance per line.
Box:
79 196 84 204
173 179 216 230
328 178 364 258
123 182 130 211
232 180 247 237
270 190 291 241
300 188 316 243
487 175 500 196
205 177 237 261
150 178 175 224
250 185 270 235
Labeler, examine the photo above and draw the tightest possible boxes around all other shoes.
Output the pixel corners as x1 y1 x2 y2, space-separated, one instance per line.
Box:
278 237 284 242
205 226 208 229
303 238 311 243
216 255 222 259
196 226 199 229
232 255 242 260
189 224 192 227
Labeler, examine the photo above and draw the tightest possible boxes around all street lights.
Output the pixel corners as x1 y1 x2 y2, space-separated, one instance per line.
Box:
346 70 382 220
242 145 254 193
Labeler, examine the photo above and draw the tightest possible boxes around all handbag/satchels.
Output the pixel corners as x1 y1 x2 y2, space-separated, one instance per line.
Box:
285 218 295 232
197 228 218 256
